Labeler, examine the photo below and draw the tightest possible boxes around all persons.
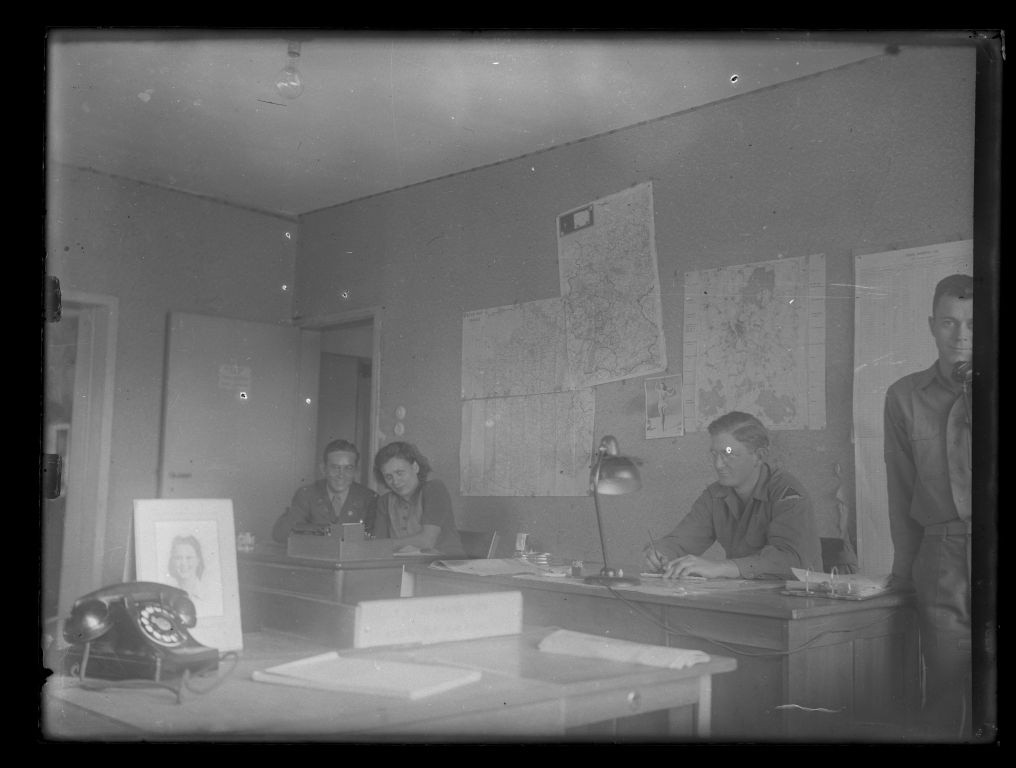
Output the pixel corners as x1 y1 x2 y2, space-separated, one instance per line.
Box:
163 535 204 591
641 411 823 582
884 274 972 741
272 438 468 561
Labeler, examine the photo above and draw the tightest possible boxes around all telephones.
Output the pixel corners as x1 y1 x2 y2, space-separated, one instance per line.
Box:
64 581 220 681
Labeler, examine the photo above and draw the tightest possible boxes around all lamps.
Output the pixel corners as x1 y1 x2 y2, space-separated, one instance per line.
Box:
584 435 642 587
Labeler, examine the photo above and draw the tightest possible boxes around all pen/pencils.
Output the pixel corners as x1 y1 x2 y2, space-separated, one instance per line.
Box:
647 529 658 559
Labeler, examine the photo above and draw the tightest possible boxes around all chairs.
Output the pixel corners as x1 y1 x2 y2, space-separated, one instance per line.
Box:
355 591 524 647
458 531 499 560
821 537 853 574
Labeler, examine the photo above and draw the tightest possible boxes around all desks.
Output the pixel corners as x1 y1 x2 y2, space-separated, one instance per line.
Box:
404 563 921 743
237 553 445 642
46 625 737 739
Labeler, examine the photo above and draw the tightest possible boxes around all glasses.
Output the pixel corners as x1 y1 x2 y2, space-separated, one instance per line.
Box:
709 450 756 463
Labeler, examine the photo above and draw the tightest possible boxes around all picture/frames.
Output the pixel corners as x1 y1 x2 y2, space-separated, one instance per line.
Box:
133 498 242 652
